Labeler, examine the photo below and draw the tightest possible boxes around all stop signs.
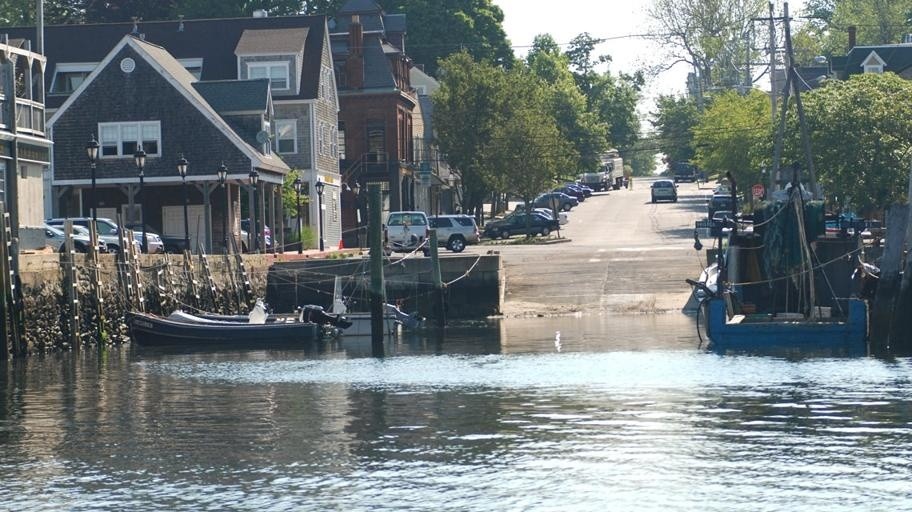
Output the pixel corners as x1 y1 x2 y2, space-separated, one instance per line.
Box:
752 184 764 197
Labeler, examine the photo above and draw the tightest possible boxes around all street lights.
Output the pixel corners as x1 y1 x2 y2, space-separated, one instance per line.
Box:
135 144 146 226
175 152 191 254
217 158 228 254
352 179 364 255
316 179 326 252
249 166 262 253
85 132 100 223
294 173 304 254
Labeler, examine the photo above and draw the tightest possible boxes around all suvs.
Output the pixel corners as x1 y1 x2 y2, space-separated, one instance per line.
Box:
45 218 165 255
381 210 431 257
707 191 738 218
650 179 680 204
426 212 480 253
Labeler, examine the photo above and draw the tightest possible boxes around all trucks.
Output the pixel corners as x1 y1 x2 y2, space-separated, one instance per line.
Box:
580 156 624 192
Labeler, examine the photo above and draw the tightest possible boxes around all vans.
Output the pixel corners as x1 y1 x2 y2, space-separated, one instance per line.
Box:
674 158 697 182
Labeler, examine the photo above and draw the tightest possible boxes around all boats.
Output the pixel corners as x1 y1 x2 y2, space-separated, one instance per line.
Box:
122 305 352 348
170 303 427 337
685 1 889 352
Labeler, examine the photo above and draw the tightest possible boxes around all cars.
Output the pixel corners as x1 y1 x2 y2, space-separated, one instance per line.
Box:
484 182 591 242
241 218 276 252
52 225 142 253
18 225 107 255
